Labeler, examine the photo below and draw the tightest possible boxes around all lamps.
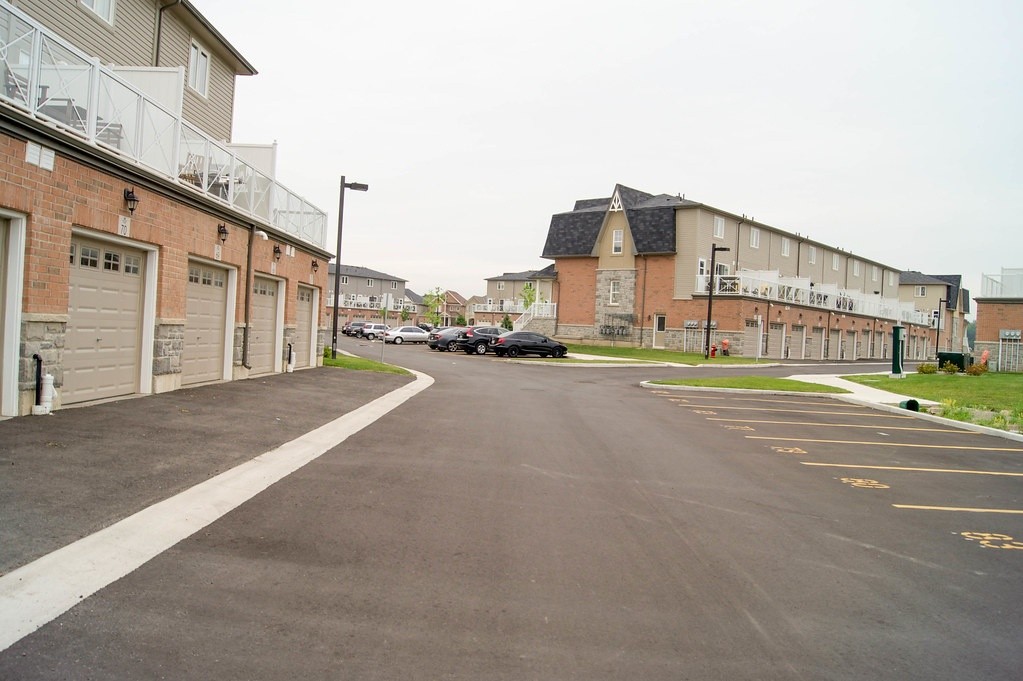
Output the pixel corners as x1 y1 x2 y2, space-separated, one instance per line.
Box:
219 222 228 244
125 186 139 216
273 245 282 263
311 258 319 273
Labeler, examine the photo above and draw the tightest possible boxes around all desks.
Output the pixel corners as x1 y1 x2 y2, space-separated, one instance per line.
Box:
24 105 103 129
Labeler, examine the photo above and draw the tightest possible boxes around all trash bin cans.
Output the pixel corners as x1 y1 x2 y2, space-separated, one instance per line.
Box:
937 351 971 373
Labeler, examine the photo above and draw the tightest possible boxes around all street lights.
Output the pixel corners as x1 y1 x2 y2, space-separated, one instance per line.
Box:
704 243 731 359
935 298 950 361
331 175 369 359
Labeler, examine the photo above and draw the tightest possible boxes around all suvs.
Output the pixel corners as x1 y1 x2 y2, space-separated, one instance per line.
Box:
360 323 391 340
456 325 510 355
345 322 368 339
427 327 452 350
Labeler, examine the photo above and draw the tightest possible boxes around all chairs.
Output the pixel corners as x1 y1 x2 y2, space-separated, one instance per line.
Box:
5 71 74 126
179 153 213 184
73 120 123 149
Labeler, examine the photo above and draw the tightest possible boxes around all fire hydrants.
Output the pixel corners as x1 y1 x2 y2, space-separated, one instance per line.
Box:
710 345 718 358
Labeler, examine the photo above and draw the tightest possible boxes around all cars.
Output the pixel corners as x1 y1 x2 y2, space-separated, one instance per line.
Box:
378 326 430 345
487 330 568 358
435 327 467 352
